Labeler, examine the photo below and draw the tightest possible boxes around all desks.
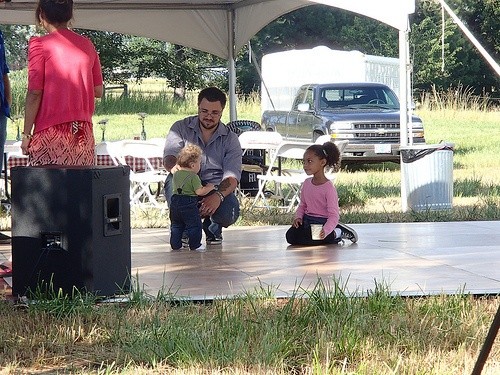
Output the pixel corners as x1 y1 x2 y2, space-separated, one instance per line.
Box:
1 138 171 205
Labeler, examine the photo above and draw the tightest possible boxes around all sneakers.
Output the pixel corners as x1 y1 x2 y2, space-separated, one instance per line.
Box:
336 221 358 245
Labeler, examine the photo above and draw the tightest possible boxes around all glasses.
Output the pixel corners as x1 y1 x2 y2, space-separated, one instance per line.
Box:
198 104 222 119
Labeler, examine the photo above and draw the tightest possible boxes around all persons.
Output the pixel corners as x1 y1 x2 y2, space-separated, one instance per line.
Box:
286 142 358 246
0 0 104 278
168 144 215 251
0 30 12 180
163 87 242 245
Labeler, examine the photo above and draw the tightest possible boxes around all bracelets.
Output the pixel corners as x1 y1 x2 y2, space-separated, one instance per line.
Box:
21 132 31 139
216 191 225 202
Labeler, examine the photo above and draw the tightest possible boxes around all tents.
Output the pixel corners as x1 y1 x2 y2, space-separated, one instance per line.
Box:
0 0 416 216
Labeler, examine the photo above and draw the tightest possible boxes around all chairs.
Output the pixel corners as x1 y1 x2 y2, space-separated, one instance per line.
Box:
249 136 324 214
328 136 347 182
106 138 169 216
356 95 371 104
236 129 284 212
321 97 329 107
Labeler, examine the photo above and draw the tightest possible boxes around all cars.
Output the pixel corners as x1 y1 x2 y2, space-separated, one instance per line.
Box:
198 63 229 81
104 67 132 79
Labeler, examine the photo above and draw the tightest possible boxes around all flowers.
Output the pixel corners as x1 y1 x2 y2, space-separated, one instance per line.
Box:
137 110 148 132
97 117 110 142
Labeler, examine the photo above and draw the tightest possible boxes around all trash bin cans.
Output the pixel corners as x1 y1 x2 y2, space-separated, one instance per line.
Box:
398 143 455 211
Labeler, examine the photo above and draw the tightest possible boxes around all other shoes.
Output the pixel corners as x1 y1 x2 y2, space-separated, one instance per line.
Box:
181 237 189 248
205 233 223 245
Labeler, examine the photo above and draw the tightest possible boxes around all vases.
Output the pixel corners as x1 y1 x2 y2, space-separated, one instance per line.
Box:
141 132 147 140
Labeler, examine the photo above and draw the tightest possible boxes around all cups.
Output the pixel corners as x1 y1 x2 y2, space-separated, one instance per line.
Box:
310 224 324 240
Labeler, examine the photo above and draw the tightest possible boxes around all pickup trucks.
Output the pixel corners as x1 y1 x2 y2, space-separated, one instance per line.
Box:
261 82 426 172
